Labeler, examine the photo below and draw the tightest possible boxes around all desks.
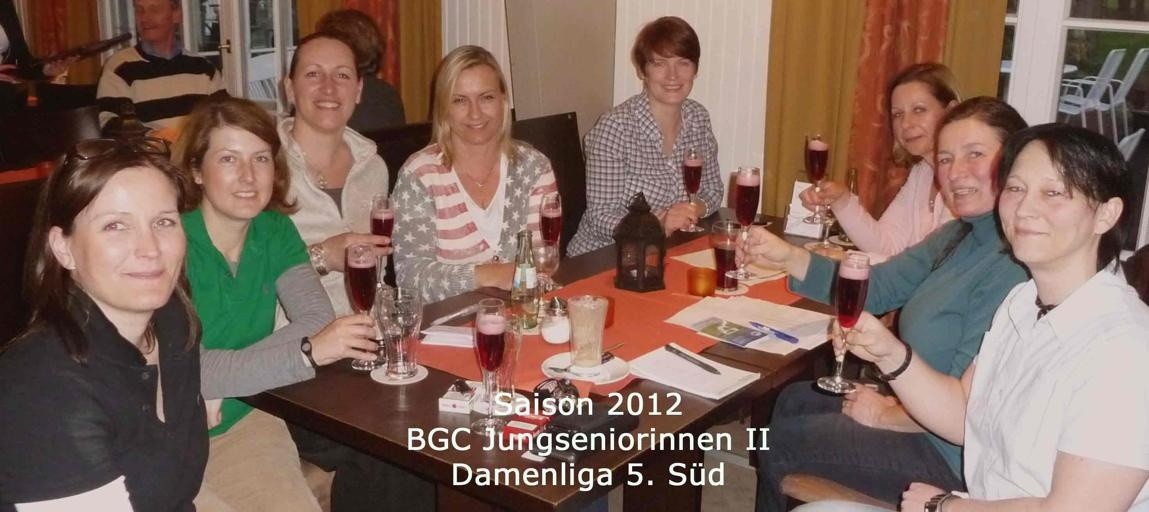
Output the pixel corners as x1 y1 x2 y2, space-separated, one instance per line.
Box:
999 59 1078 104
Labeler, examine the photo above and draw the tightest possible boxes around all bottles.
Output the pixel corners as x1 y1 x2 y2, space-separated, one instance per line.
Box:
838 168 860 242
510 231 538 328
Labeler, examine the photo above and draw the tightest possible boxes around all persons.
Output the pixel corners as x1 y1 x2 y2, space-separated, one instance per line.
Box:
276 34 434 510
178 98 380 512
567 16 723 262
798 64 959 382
2 138 209 510
736 96 1031 512
387 44 561 306
794 123 1148 512
96 0 229 167
316 8 407 193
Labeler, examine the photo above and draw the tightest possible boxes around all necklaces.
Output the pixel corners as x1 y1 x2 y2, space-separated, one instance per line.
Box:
292 133 341 189
451 154 500 191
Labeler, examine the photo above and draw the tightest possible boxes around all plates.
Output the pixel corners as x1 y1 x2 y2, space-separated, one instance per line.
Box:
540 352 630 386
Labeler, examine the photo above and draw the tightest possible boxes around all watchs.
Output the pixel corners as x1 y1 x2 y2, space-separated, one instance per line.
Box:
311 242 329 274
302 336 318 373
873 342 912 382
925 492 959 511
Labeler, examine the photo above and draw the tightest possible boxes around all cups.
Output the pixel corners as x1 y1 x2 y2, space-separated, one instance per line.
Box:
567 294 608 377
709 220 741 293
801 131 833 226
678 149 705 233
815 251 871 396
532 240 560 309
538 193 563 290
342 242 379 371
470 297 507 439
724 166 762 281
375 286 424 381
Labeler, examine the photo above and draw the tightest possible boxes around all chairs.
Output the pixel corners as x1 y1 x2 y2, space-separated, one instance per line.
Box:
1117 128 1146 162
1063 48 1149 146
353 119 436 191
514 110 588 266
1058 48 1127 135
0 160 57 341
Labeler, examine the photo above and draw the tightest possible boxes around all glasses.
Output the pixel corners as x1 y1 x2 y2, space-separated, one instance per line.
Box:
62 134 171 189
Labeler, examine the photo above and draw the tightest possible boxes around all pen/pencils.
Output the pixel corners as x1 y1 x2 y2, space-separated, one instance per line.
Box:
430 303 479 326
664 344 721 375
748 320 799 344
